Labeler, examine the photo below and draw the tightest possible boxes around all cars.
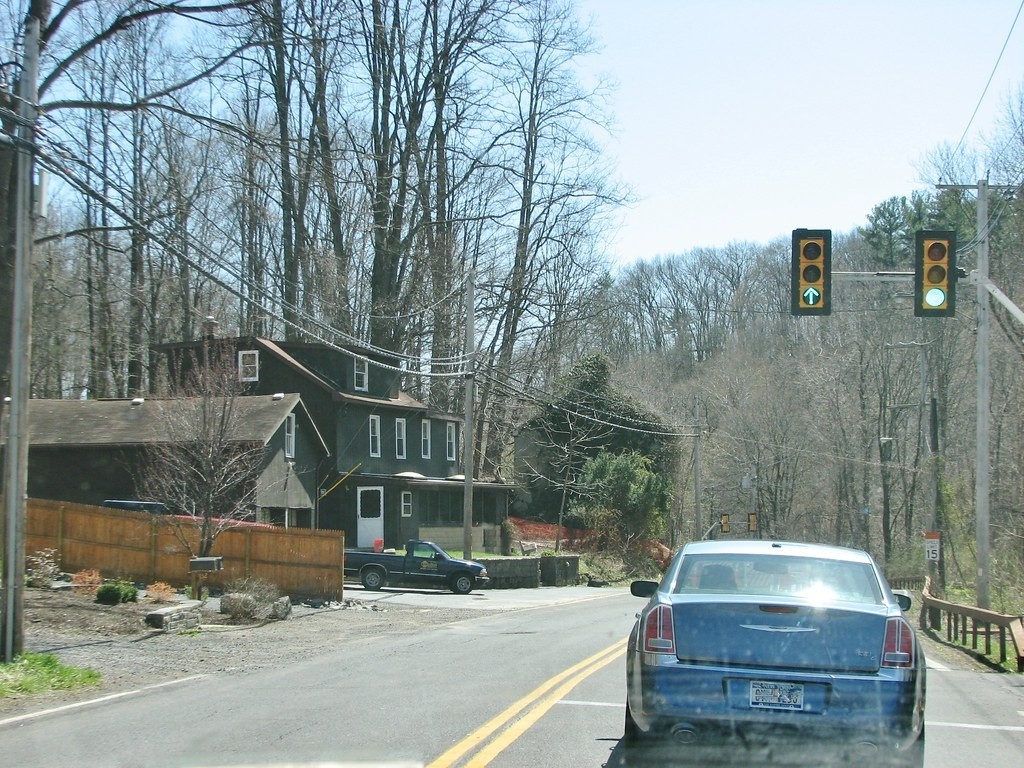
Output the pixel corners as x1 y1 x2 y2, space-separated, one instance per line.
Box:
624 539 927 768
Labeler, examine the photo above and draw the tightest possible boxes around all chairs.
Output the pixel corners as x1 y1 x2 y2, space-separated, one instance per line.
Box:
699 564 740 592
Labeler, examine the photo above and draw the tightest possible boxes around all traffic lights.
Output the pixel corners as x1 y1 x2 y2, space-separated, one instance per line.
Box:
914 231 956 317
791 228 832 316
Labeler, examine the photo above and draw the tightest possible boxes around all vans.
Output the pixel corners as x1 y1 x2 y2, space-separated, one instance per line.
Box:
104 500 174 515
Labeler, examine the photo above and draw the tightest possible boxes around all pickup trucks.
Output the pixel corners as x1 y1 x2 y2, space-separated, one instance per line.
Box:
344 539 490 595
